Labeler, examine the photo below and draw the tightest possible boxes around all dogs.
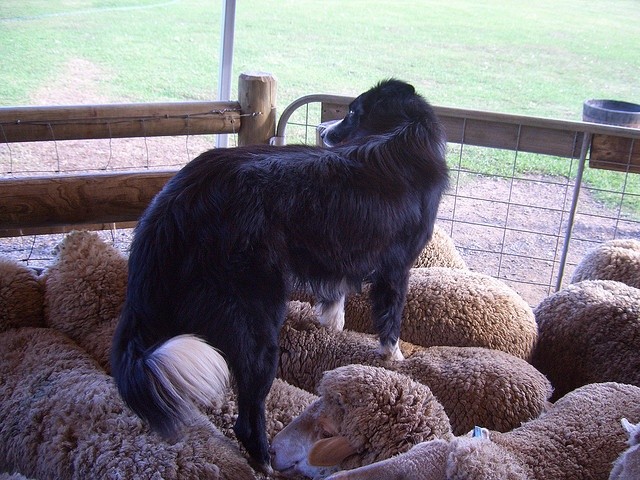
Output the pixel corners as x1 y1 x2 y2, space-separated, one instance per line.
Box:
111 77 454 480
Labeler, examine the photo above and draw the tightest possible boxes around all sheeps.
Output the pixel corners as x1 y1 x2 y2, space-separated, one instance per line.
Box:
401 221 466 268
211 365 459 480
536 278 640 382
1 332 255 480
277 300 554 434
569 238 639 290
43 230 131 374
0 258 41 330
341 268 540 363
331 381 640 480
605 416 638 480
